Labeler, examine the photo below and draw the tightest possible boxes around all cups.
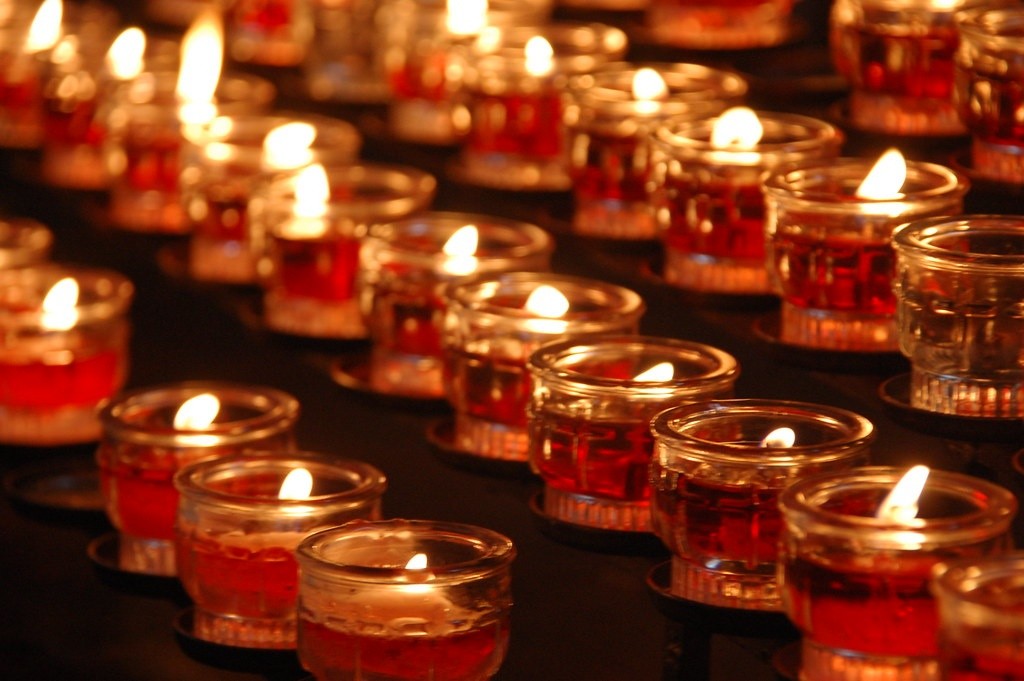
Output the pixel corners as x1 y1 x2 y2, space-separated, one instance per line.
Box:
890 213 1024 418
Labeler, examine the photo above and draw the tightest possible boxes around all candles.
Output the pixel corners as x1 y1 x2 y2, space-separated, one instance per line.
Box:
0 0 1024 681
